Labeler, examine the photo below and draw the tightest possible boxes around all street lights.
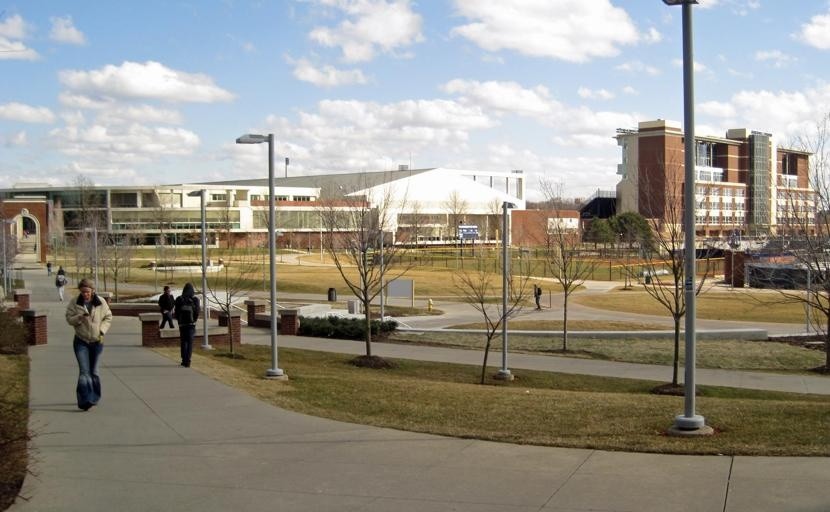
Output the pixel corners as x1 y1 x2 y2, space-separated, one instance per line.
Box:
188 189 209 346
503 202 518 369
84 226 97 292
3 219 16 296
237 133 278 371
663 0 699 416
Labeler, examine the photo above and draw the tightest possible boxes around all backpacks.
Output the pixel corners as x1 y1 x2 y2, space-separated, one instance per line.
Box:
180 298 196 324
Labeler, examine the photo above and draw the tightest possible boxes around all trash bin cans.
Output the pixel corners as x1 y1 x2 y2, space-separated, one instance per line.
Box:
348 300 360 314
219 314 228 326
646 275 651 284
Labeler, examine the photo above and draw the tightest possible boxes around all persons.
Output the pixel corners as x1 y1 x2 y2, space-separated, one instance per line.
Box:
56 272 68 302
65 278 114 410
157 286 175 328
58 266 64 273
47 261 52 276
172 282 201 368
533 284 541 311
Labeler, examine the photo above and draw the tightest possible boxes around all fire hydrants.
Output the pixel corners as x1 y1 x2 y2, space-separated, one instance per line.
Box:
428 299 433 312
